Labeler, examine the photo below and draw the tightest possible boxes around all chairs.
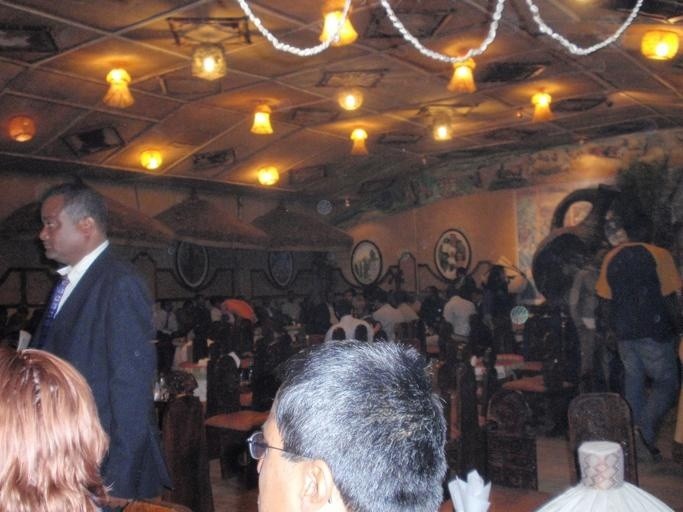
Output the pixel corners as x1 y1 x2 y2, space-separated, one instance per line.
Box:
89 337 641 510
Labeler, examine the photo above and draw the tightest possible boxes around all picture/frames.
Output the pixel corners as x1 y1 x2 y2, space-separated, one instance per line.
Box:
267 246 294 288
349 239 382 287
174 240 208 289
432 227 471 282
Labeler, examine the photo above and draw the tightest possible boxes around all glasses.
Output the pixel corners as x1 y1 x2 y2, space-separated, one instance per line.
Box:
247 431 294 461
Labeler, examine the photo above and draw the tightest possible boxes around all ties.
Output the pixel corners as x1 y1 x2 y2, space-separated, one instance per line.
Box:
38 277 68 348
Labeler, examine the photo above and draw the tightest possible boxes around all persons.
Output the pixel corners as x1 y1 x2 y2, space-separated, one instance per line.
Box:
0 263 683 457
26 184 174 511
0 345 117 511
595 215 683 456
245 336 450 512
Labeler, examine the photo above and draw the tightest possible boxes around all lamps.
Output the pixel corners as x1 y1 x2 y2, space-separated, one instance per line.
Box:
348 126 370 156
189 42 227 80
246 101 276 138
7 113 34 142
430 110 455 143
445 57 477 94
529 87 555 124
139 147 165 170
317 4 359 47
100 67 135 108
637 29 680 60
337 88 364 111
256 163 280 186
